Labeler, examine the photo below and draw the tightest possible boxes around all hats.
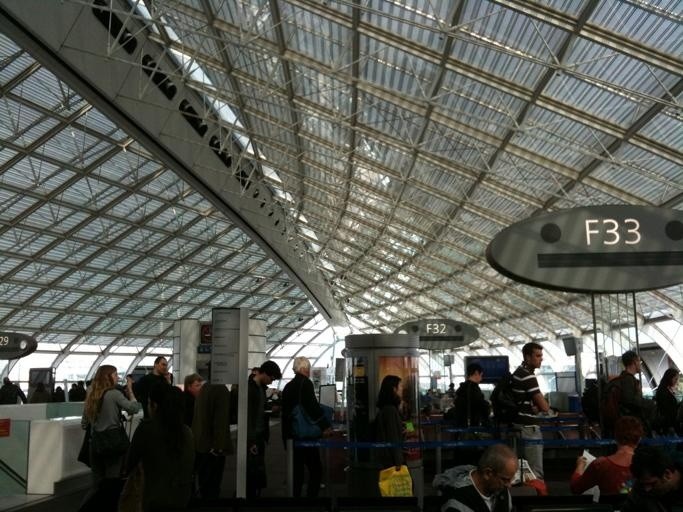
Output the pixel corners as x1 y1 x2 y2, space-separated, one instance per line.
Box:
260 359 282 380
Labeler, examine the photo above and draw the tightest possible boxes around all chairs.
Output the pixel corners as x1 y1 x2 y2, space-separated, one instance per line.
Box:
416 413 601 449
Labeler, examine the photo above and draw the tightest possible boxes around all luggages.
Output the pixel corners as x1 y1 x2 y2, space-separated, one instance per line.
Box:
318 427 347 483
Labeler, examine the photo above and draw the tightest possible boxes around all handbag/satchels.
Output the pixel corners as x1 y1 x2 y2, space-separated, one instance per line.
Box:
364 406 402 464
378 464 413 497
117 460 146 512
77 423 93 468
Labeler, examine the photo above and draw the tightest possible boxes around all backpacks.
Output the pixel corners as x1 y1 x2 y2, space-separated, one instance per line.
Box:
580 376 605 423
489 367 520 424
600 373 634 425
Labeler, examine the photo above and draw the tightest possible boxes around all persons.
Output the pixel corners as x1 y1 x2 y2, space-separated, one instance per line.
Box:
376 342 683 511
81 355 283 510
1 377 27 404
30 381 92 403
281 357 334 496
